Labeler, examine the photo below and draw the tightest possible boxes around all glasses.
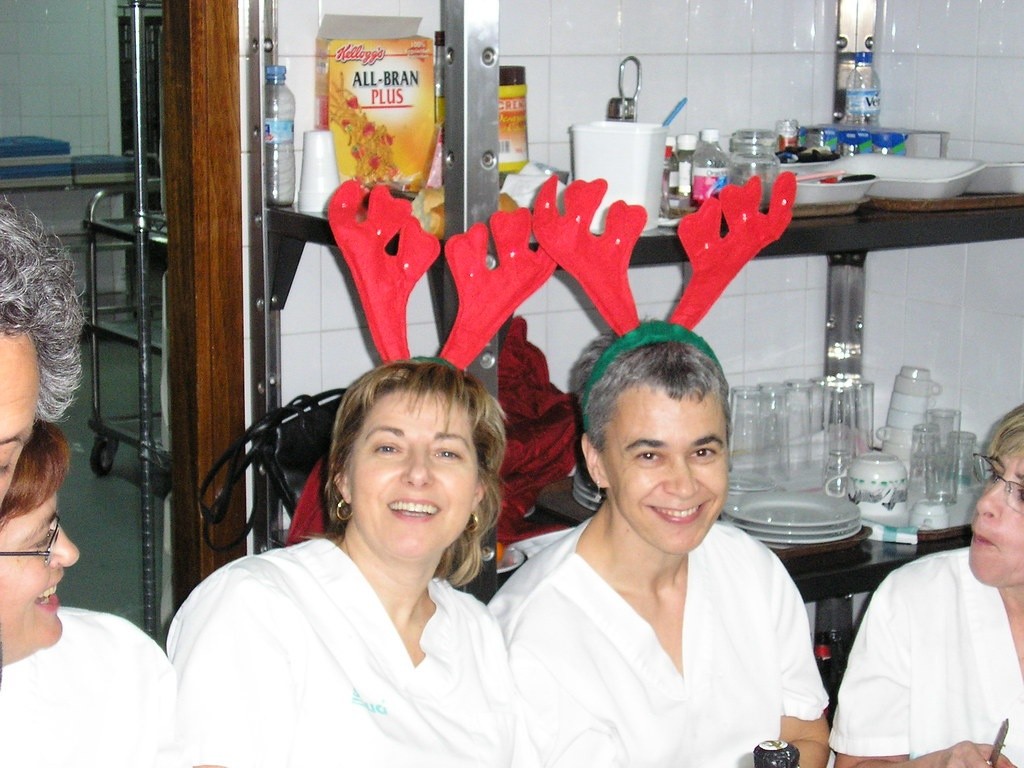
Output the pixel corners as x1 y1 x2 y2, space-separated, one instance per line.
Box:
971 453 1024 519
0 512 67 568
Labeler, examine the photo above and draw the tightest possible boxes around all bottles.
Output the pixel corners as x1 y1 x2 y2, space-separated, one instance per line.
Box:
779 119 909 155
814 631 847 730
434 30 446 123
265 66 294 205
846 52 880 126
498 65 529 172
659 130 781 219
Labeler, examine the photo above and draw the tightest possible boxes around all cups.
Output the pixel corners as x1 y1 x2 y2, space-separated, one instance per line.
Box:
297 131 339 212
732 366 977 530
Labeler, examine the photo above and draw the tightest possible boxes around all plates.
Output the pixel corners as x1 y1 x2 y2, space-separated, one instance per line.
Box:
496 547 525 573
572 469 606 511
720 491 862 544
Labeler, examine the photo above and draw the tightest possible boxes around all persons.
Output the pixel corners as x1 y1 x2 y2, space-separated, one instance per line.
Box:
828 403 1024 768
0 418 178 768
0 202 84 507
486 319 828 768
166 358 541 768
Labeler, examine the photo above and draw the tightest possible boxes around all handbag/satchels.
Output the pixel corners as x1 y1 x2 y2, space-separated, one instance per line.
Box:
198 393 343 553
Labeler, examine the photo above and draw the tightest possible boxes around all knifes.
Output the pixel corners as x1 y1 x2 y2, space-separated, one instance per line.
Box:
986 718 1009 768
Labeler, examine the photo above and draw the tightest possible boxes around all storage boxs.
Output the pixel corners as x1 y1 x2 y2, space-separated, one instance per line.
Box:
313 12 437 193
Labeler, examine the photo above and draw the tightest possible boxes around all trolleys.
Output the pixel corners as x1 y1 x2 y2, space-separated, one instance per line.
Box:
82 182 160 476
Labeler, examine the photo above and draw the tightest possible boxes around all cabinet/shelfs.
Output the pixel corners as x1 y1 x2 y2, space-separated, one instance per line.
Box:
78 182 175 501
0 151 162 319
245 0 1024 603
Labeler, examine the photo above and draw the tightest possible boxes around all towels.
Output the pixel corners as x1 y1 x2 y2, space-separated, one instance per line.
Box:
282 315 583 548
